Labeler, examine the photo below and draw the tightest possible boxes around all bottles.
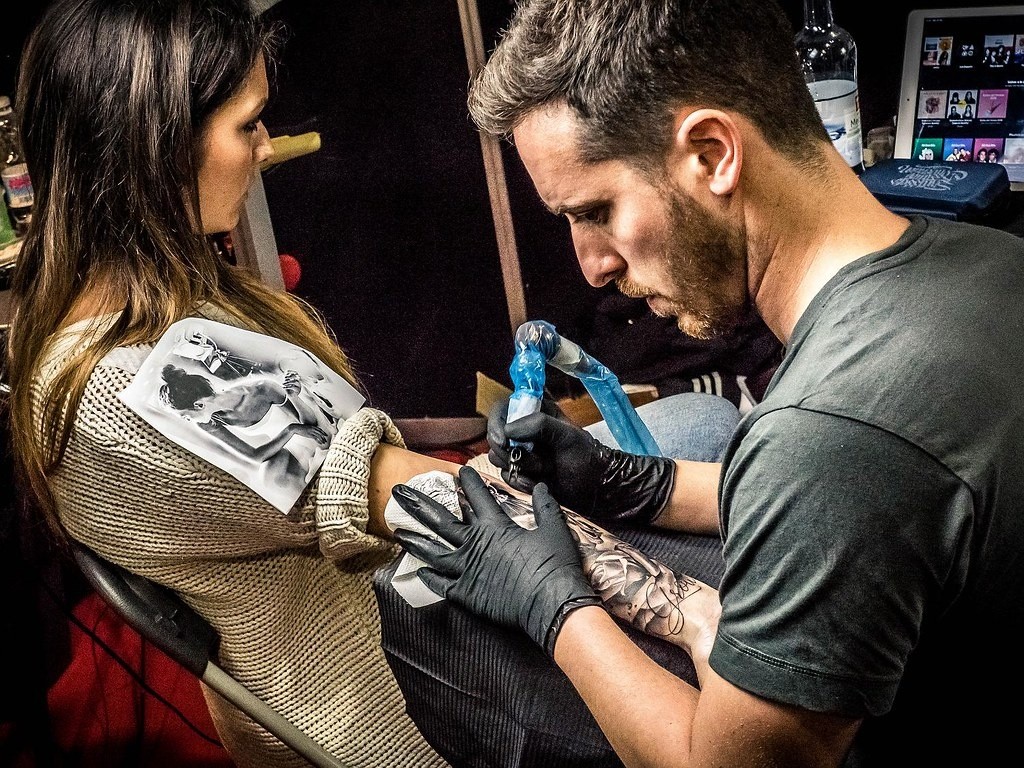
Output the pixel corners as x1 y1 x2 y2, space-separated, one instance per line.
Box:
0 95 43 238
788 0 864 178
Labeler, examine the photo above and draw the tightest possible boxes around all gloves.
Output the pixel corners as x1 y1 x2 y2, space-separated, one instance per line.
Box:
485 392 676 530
389 465 602 662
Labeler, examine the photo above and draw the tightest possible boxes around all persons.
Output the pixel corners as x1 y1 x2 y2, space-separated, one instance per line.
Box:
392 1 1024 766
983 38 1024 66
920 148 999 163
938 51 948 65
948 92 975 119
0 2 720 768
159 365 332 485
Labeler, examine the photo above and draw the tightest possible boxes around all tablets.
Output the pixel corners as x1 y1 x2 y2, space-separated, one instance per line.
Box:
889 5 1024 190
